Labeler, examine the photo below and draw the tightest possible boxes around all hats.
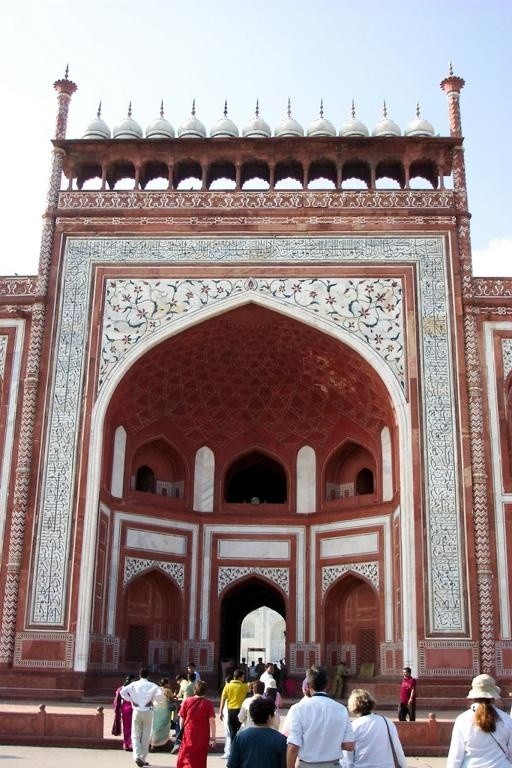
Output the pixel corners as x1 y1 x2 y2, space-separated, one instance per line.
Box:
267 678 276 688
466 674 501 700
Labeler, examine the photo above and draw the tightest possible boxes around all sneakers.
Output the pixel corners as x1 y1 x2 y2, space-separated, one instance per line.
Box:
171 743 180 754
221 756 229 759
123 746 156 767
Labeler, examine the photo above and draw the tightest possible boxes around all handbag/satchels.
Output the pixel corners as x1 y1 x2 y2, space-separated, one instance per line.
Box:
167 699 181 711
177 727 184 741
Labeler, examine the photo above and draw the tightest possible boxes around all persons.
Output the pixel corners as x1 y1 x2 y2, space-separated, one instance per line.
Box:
398 667 416 721
112 658 404 767
447 674 512 767
